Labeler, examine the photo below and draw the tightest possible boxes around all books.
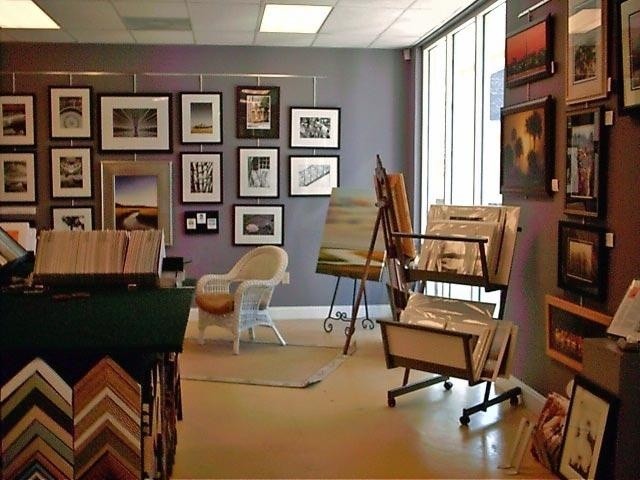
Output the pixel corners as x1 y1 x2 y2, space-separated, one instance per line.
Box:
0 226 35 287
0 222 37 289
32 229 165 285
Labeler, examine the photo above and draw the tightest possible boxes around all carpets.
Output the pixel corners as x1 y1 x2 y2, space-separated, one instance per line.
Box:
179 336 357 389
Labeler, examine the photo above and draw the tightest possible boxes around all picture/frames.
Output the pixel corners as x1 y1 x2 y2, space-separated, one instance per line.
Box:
236 145 281 199
231 203 286 247
614 0 640 117
562 103 608 224
557 374 621 480
315 188 384 282
100 160 174 247
288 154 340 197
179 151 224 205
0 219 29 266
47 85 94 141
373 173 416 260
0 149 39 207
544 294 614 374
564 0 608 106
96 91 174 154
236 85 280 138
400 290 519 382
499 94 555 200
179 91 223 145
0 92 37 149
50 206 96 232
504 12 555 89
29 220 37 254
184 210 220 235
416 204 521 286
288 105 341 150
47 145 95 200
557 220 609 303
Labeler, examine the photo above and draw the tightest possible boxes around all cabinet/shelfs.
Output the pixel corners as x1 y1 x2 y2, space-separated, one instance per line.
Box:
343 169 522 428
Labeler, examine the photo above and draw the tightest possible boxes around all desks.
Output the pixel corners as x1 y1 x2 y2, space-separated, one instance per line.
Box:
1 278 195 480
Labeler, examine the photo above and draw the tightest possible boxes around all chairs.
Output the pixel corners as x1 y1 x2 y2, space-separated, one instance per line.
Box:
193 244 290 356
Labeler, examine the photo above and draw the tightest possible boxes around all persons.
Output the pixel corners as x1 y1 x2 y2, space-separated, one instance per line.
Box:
570 419 593 474
